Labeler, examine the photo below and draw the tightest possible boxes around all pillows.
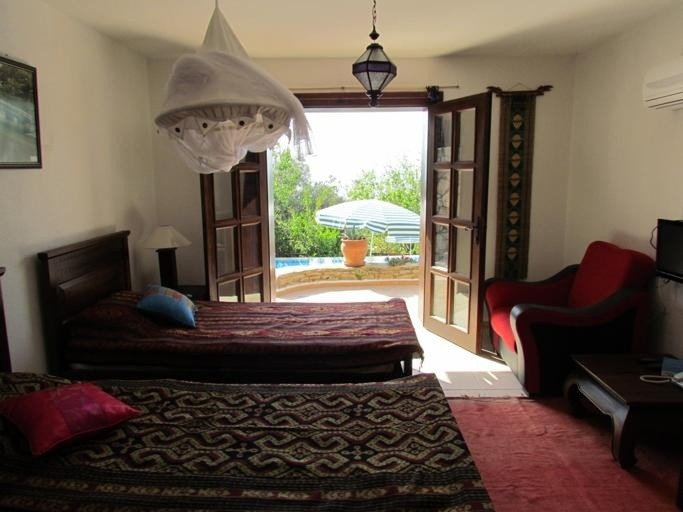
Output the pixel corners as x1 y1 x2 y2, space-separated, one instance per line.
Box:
1 382 141 455
135 281 199 329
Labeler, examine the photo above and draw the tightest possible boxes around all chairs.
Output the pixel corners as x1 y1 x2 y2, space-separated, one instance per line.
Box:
482 240 654 397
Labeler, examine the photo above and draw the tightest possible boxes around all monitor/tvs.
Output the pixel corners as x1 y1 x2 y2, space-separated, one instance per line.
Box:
655 218 683 283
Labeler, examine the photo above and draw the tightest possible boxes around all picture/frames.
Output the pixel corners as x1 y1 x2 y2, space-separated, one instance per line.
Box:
0 55 43 168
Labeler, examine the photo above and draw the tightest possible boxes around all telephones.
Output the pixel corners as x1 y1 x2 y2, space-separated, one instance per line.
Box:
672 371 682 388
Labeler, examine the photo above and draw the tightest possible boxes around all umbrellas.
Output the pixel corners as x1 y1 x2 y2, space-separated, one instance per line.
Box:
315 198 420 260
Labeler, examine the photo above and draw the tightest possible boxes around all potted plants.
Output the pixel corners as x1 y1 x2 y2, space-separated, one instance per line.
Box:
337 218 369 266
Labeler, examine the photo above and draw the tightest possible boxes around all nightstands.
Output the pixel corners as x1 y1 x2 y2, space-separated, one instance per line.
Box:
169 285 207 301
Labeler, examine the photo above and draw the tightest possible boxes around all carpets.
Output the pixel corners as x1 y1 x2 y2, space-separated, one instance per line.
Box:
445 396 683 511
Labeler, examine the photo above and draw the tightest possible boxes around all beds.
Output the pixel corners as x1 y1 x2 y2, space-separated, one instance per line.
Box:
0 267 492 512
37 230 425 384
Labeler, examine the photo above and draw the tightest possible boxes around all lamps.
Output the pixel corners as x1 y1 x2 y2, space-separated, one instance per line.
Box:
352 0 397 108
141 224 191 288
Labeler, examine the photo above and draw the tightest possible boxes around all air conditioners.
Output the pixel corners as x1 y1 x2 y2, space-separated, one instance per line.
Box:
643 55 683 112
